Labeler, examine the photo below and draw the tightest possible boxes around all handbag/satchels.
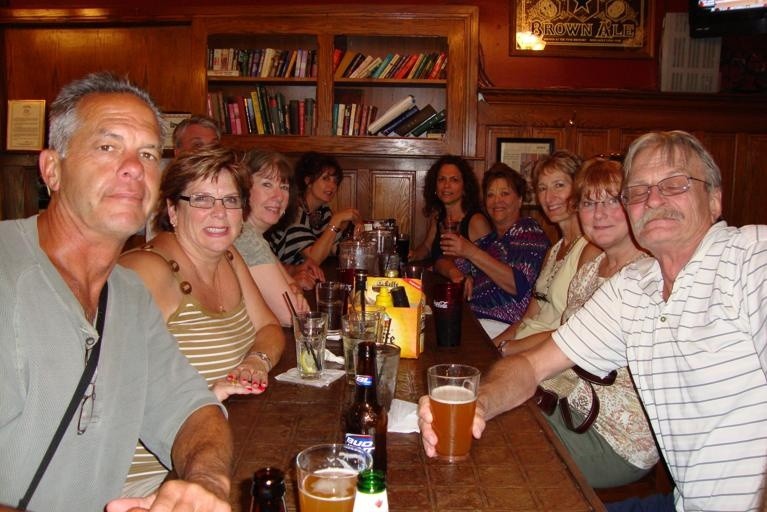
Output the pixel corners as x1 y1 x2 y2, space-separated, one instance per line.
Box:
496 319 580 399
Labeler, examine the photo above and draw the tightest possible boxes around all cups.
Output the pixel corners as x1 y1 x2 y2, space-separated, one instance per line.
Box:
338 219 423 289
352 341 401 400
315 280 342 342
439 219 461 237
341 310 384 393
425 363 481 464
431 281 464 348
295 443 368 512
293 312 331 380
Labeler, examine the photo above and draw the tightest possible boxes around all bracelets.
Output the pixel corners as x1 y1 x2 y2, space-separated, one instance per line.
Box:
326 223 342 234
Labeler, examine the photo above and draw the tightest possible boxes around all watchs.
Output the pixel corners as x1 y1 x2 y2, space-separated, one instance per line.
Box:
497 339 512 354
247 352 273 374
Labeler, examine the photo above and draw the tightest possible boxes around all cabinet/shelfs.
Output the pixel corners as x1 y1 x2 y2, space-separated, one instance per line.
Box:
202 4 480 159
320 157 432 252
479 87 767 249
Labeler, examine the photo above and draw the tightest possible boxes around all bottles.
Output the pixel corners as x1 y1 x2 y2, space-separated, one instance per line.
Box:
419 303 425 353
351 271 411 308
353 466 389 512
344 341 389 470
248 465 288 512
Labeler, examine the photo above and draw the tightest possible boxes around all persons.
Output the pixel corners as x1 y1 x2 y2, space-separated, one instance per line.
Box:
537 152 661 488
263 152 362 266
145 116 223 246
439 161 553 338
232 148 313 329
406 153 492 260
0 75 235 512
116 143 286 500
491 147 604 402
416 129 767 511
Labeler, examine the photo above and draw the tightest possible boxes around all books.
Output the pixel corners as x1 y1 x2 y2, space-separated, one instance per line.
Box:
207 45 447 139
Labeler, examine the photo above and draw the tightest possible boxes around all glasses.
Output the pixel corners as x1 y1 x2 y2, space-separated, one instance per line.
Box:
621 175 711 205
176 194 247 209
573 196 622 212
78 348 98 435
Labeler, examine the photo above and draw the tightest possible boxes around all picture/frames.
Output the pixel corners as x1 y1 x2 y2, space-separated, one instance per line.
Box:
508 1 655 63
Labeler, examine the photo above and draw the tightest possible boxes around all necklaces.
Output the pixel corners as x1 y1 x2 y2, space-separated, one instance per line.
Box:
199 283 228 314
546 232 583 284
